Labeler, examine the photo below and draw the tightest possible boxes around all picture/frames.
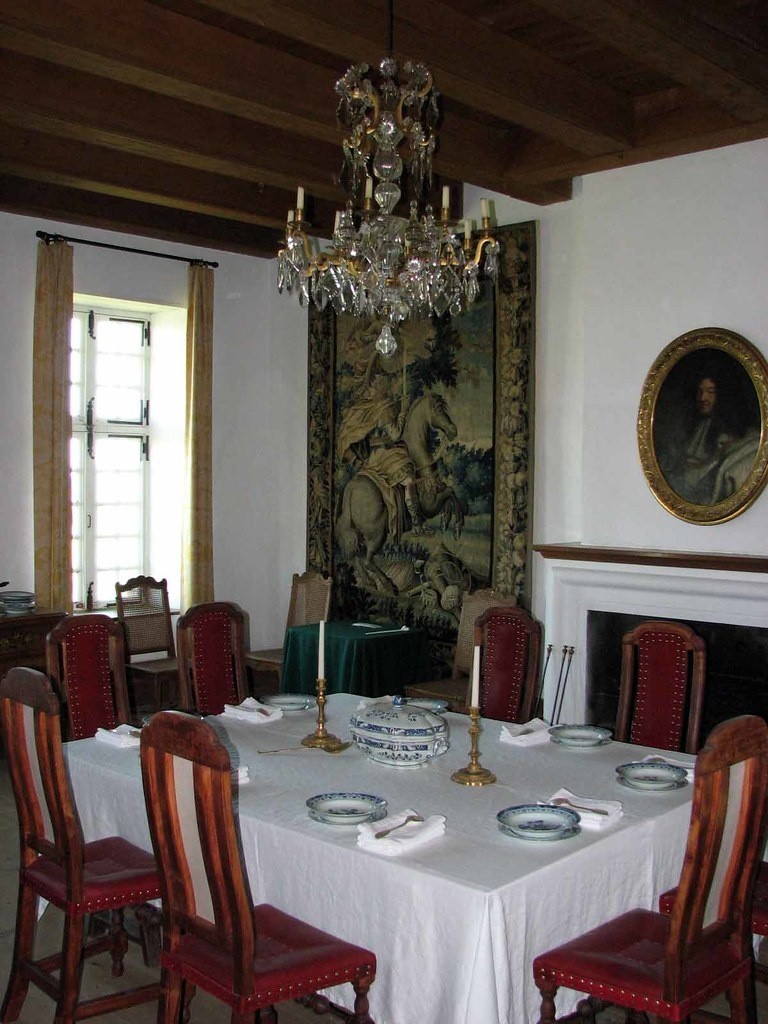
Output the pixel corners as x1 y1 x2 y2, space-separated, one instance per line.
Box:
635 327 768 527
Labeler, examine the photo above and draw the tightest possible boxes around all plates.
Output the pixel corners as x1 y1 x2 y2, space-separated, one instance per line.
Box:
307 811 386 825
431 708 447 715
266 703 317 715
550 736 612 748
498 823 581 841
616 776 688 792
0 591 35 614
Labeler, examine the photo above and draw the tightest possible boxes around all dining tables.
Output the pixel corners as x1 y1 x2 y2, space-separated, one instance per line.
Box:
37 693 745 1024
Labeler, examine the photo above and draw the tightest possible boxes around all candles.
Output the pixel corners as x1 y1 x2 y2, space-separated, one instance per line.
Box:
318 620 324 678
471 646 480 707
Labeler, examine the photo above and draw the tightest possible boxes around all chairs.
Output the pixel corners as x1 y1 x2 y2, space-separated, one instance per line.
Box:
246 571 332 698
405 594 516 715
140 711 366 1024
532 715 768 1024
471 606 545 724
114 575 191 720
613 619 704 754
659 859 768 1024
45 614 132 742
176 601 246 715
0 667 195 1024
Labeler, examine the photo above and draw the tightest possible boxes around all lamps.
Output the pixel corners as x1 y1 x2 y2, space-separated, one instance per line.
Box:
275 0 498 359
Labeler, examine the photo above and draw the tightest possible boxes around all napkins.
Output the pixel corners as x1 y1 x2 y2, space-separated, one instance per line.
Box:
220 697 285 725
94 723 141 749
498 717 561 747
632 754 695 785
356 809 446 857
536 787 624 829
238 765 250 786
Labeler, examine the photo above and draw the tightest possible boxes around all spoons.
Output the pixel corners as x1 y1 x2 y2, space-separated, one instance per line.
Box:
549 799 608 815
375 815 424 838
111 728 140 738
237 705 270 717
647 758 694 770
258 740 353 753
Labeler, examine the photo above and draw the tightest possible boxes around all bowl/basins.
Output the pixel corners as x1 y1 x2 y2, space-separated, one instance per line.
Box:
306 792 388 823
402 698 448 711
548 726 612 745
497 804 581 836
348 695 450 767
615 763 687 788
260 693 316 711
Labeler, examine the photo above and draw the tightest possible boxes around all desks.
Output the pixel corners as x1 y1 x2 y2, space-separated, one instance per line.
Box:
73 605 182 621
276 620 425 698
